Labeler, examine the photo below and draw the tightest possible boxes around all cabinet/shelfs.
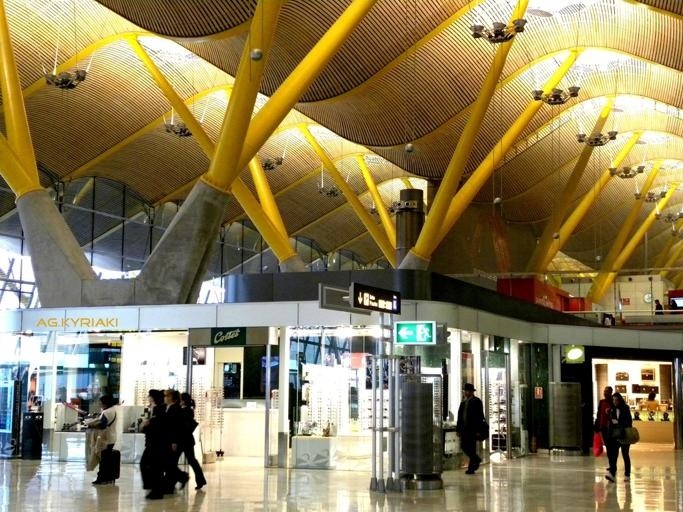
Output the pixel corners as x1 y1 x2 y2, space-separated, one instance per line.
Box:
493 383 516 458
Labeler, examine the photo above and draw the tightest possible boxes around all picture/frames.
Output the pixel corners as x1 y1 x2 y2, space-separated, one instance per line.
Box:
641 368 655 380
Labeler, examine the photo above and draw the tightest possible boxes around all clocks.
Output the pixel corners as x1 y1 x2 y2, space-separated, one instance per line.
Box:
643 293 654 303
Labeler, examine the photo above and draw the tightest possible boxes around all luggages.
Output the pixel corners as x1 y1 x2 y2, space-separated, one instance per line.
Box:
99 448 120 482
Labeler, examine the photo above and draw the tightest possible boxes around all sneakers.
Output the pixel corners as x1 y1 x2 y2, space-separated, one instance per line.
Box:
606 473 615 482
465 457 483 474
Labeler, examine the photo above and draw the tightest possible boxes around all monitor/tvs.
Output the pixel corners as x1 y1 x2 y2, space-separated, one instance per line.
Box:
182 346 206 366
32 395 43 407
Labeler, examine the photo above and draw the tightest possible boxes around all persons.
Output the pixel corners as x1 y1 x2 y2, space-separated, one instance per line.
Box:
644 392 661 411
83 392 119 484
604 391 633 486
592 386 614 469
668 299 678 325
454 382 490 475
653 299 665 326
136 388 208 500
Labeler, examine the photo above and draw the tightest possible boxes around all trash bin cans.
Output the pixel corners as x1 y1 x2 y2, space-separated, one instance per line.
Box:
21 411 43 460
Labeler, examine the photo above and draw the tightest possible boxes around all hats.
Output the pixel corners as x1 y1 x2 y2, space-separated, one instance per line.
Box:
462 382 476 392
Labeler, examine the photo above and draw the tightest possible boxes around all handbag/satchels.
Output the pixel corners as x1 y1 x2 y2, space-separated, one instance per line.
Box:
593 432 603 457
625 427 640 443
477 419 489 440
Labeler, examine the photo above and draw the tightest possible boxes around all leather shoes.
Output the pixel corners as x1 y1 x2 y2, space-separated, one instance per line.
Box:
145 471 206 500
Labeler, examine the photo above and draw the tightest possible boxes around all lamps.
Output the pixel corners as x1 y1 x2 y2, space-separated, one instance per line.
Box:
25 0 417 213
468 0 683 236
